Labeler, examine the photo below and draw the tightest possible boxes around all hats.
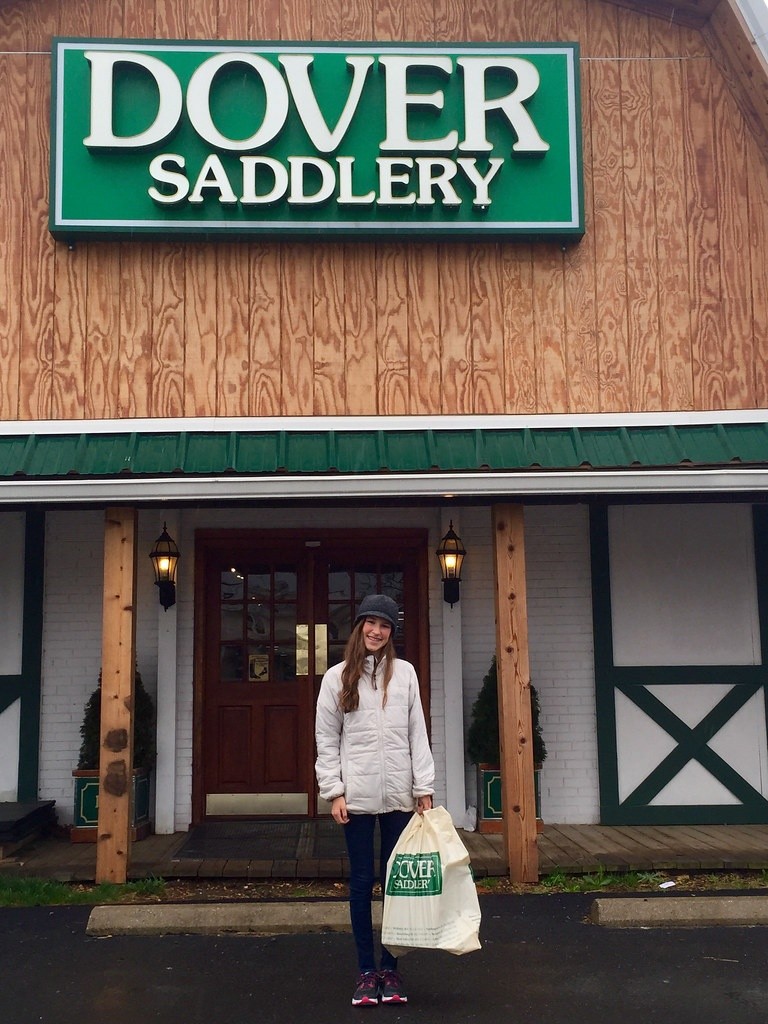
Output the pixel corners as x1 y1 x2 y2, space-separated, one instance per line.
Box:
353 595 398 639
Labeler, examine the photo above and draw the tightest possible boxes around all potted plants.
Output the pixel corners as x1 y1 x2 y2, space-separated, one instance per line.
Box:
70 651 156 843
467 655 547 835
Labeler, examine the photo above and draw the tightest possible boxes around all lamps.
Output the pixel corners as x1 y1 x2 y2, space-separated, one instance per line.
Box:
435 518 466 609
149 521 181 611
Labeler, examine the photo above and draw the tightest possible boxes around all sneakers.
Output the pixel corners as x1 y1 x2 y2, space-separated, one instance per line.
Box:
351 972 380 1004
380 969 407 1003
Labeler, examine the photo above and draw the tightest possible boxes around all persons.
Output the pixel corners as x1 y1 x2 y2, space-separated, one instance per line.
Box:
314 594 437 1005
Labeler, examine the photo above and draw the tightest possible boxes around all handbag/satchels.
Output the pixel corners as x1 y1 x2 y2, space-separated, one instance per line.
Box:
382 805 482 958
